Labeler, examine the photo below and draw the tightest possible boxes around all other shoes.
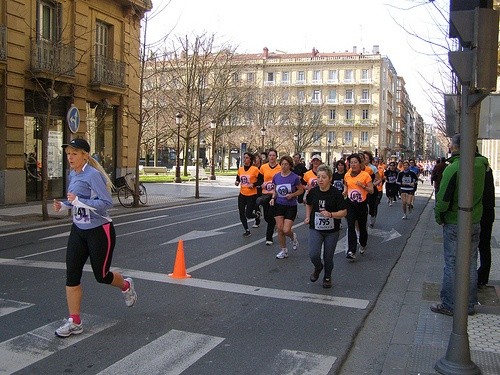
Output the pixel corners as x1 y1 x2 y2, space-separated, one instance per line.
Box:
478 281 487 289
389 204 392 207
430 304 454 316
368 224 373 228
402 216 407 219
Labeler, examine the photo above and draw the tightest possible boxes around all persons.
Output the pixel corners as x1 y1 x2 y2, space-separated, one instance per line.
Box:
409 159 419 208
92 151 113 176
253 152 268 228
302 163 348 288
474 144 496 289
23 152 36 181
429 132 485 317
293 153 350 229
355 151 404 245
258 149 283 246
396 161 416 220
269 155 304 258
342 154 374 259
52 139 138 338
432 157 448 209
235 152 263 238
415 160 438 185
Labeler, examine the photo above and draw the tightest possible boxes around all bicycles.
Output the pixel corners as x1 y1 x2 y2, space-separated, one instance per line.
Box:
114 172 147 208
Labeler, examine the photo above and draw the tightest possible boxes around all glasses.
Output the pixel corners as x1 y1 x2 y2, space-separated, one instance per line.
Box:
389 165 395 166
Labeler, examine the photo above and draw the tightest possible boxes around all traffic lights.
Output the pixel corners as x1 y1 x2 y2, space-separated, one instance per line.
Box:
446 8 497 92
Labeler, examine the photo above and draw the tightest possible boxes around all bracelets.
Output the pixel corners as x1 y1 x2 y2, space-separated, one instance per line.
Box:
363 185 366 189
329 212 332 218
271 195 275 199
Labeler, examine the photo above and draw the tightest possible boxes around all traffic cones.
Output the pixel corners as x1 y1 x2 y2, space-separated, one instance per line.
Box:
169 241 192 279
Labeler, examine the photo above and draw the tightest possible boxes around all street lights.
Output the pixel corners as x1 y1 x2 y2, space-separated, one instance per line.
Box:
174 110 183 184
342 143 346 154
260 126 266 153
208 119 217 180
327 140 332 166
294 134 298 153
353 144 358 154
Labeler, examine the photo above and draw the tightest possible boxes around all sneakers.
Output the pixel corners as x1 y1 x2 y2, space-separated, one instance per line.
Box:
310 264 323 282
253 224 259 227
266 241 273 245
255 212 260 225
346 252 356 259
322 276 331 288
276 248 289 259
359 243 367 253
243 231 251 237
121 278 137 306
55 318 83 336
290 233 299 251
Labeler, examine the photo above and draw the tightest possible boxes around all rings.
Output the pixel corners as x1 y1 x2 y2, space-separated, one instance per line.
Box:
68 198 69 200
323 214 325 216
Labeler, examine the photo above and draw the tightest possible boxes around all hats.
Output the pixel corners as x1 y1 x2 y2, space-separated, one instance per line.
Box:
61 139 90 153
310 154 322 161
